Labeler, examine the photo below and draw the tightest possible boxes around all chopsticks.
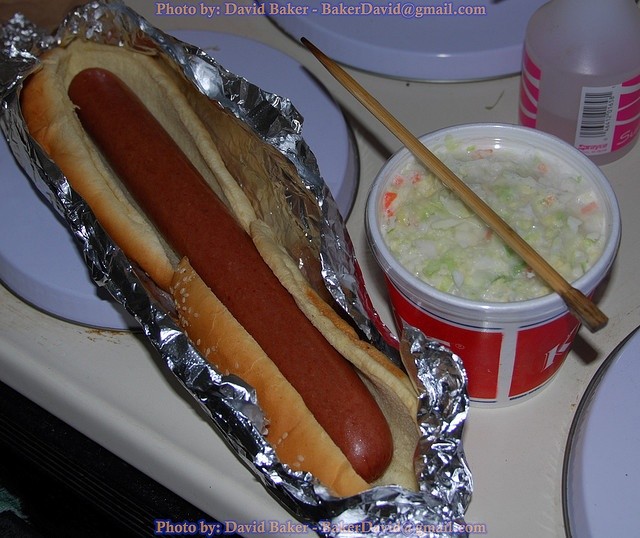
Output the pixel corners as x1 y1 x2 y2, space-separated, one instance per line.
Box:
301 37 609 333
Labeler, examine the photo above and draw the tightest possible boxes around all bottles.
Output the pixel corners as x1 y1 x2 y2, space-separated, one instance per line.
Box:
520 2 639 159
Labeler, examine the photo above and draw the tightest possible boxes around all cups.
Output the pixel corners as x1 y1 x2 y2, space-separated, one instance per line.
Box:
365 122 622 406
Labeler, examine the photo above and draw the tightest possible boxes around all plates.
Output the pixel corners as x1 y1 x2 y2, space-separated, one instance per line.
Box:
0 30 359 333
258 0 543 84
562 329 640 537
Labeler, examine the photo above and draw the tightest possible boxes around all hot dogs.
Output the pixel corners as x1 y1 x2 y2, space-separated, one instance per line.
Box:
11 35 426 499
